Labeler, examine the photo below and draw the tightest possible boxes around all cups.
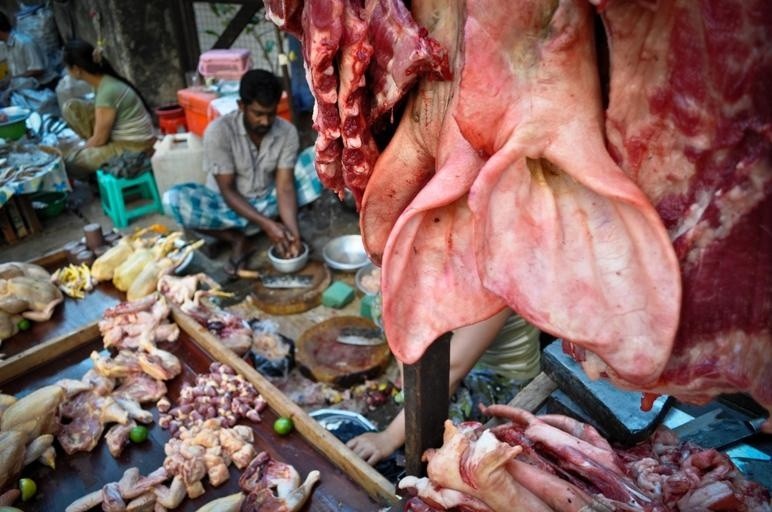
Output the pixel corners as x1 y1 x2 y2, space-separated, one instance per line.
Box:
62 223 122 266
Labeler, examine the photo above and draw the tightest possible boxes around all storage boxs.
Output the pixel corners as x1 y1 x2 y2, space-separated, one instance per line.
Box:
212 92 293 123
177 88 218 135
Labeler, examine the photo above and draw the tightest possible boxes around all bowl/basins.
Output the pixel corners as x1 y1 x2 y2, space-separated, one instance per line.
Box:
268 242 309 273
321 233 372 270
302 408 377 447
155 104 183 118
356 262 383 296
0 107 31 142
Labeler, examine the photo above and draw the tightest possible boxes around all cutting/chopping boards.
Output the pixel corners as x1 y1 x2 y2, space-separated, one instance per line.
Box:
293 315 394 388
251 261 334 315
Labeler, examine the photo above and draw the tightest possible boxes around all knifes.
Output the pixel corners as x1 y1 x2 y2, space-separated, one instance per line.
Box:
231 270 314 290
336 325 384 346
660 404 764 454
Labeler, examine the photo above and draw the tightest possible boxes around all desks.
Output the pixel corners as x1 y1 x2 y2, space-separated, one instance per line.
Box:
0 223 408 512
0 141 65 244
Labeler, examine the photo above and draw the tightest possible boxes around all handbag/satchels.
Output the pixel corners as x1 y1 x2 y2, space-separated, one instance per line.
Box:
105 146 144 179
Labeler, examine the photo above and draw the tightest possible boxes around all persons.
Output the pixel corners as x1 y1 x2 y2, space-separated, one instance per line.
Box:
0 1 62 90
343 305 542 466
160 69 327 275
60 37 157 189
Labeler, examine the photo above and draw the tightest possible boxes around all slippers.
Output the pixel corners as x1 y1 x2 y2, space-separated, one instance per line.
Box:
225 253 247 277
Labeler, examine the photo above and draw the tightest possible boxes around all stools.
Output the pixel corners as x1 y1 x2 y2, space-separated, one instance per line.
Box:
96 169 163 227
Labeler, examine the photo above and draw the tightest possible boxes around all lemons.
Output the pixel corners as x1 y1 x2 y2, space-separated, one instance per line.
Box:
129 426 148 444
273 411 295 436
17 320 28 332
18 477 37 502
